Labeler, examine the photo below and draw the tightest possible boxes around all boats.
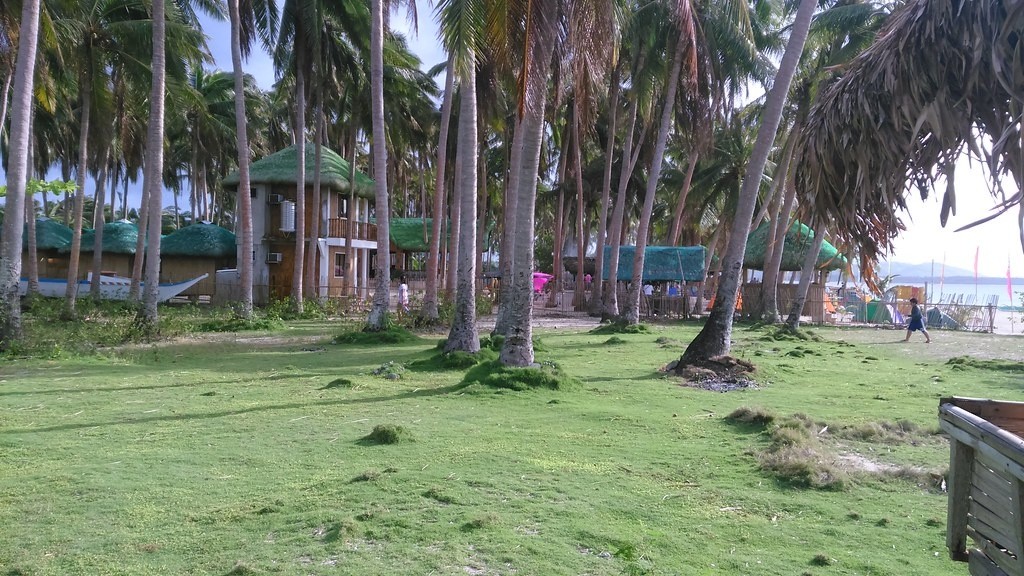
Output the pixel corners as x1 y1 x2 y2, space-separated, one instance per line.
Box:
17 272 211 304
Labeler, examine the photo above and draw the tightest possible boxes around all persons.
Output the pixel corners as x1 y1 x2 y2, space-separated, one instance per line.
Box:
585 273 592 291
396 274 409 323
618 281 695 315
904 298 931 343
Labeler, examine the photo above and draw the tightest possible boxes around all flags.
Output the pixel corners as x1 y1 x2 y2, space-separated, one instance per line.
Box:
940 267 944 287
1006 257 1011 299
974 248 978 277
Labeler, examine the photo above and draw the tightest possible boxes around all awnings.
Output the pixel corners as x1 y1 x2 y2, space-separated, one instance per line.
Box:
389 218 496 252
602 245 720 282
